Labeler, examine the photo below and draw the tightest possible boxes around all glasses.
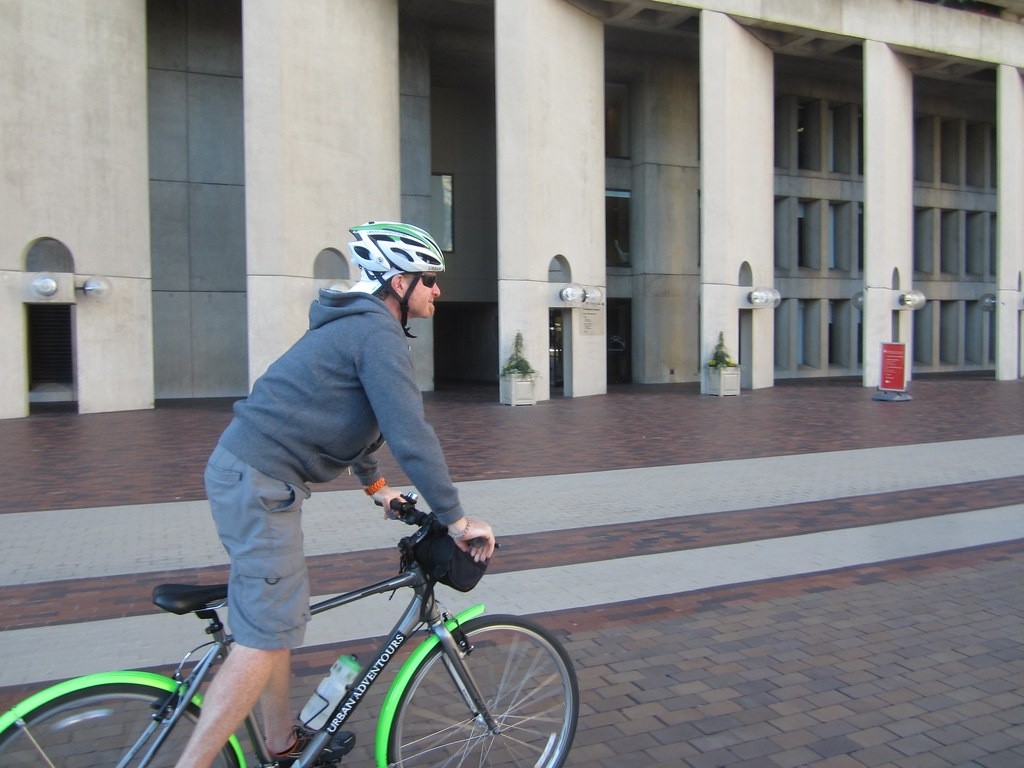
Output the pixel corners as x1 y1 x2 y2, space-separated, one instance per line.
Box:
407 272 440 288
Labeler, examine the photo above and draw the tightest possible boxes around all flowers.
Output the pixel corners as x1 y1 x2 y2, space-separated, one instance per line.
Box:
497 331 545 384
706 330 746 374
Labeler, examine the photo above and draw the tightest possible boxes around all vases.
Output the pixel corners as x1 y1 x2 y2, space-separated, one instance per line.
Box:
499 371 536 407
706 367 742 397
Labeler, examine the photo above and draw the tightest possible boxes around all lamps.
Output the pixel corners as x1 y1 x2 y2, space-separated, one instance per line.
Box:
747 286 782 310
30 272 62 299
899 290 926 310
74 276 114 301
559 284 602 309
979 294 996 312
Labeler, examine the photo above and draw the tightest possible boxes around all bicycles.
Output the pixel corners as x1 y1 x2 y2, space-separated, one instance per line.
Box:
0 491 580 768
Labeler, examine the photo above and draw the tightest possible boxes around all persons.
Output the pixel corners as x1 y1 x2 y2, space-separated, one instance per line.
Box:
173 221 493 768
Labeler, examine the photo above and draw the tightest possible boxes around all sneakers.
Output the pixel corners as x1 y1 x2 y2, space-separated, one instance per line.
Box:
262 724 356 768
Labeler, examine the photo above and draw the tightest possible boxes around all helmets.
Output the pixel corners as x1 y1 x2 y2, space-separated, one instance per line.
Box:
347 220 446 272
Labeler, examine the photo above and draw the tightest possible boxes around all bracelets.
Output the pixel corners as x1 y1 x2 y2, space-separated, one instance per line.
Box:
448 516 471 540
362 477 385 495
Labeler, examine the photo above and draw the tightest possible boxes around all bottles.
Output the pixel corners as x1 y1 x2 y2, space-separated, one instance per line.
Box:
300 654 360 730
